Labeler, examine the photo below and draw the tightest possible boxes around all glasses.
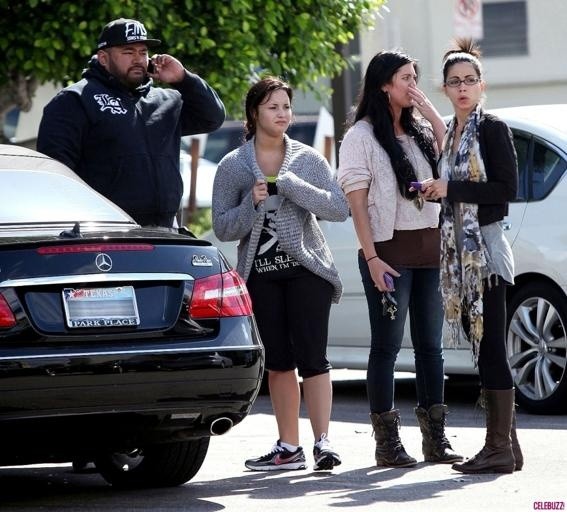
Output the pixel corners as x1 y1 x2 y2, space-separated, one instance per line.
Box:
444 78 482 87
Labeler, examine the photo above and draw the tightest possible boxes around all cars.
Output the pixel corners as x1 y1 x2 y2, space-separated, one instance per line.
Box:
158 109 338 228
0 141 266 493
201 104 565 420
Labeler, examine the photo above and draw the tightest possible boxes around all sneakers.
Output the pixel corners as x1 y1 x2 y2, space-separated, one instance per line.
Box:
313 433 342 471
245 439 308 471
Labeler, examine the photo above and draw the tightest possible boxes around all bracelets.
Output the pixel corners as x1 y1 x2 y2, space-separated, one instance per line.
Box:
366 255 378 263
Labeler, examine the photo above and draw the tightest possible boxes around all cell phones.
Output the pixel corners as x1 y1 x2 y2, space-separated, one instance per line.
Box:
384 273 394 290
410 182 423 190
147 60 155 74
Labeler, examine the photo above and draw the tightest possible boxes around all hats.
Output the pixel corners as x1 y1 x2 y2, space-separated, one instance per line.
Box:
97 18 161 51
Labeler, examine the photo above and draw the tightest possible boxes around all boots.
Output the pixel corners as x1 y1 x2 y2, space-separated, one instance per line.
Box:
415 404 464 462
369 409 418 468
452 387 523 474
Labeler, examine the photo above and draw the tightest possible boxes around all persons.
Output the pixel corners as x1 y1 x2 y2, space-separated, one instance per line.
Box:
213 76 349 472
336 50 465 470
408 34 524 475
37 17 226 228
